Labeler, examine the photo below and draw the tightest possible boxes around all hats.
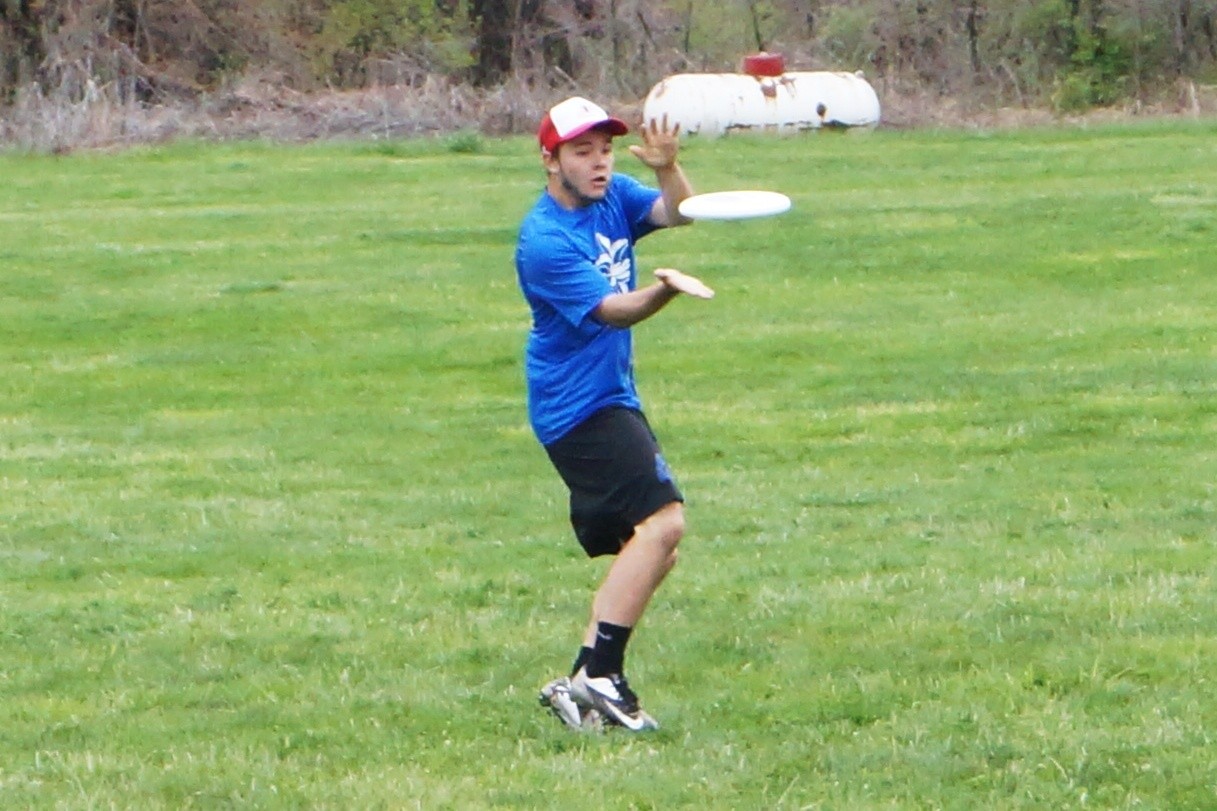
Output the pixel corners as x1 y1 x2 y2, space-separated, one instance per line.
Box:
538 95 630 151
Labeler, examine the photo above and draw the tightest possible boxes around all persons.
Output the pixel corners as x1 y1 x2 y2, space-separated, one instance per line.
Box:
513 96 714 737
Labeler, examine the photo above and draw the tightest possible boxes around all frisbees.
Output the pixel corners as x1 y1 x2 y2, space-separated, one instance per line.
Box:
675 189 794 223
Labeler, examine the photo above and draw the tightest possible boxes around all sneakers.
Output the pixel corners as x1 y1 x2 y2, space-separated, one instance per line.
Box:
571 665 658 731
541 676 606 732
